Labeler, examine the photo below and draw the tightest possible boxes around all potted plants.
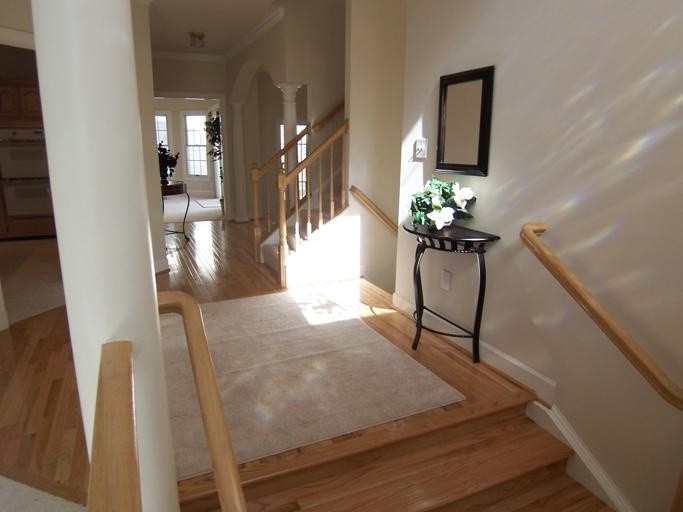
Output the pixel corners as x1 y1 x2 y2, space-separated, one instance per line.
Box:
203 109 223 213
158 140 180 184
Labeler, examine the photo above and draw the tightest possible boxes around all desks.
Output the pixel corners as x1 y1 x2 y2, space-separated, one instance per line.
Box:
402 218 500 364
161 180 190 250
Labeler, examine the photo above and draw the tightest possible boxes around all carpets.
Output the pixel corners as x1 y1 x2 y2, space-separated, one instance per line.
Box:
159 288 467 482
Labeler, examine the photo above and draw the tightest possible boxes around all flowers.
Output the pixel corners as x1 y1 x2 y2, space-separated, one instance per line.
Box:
406 175 477 231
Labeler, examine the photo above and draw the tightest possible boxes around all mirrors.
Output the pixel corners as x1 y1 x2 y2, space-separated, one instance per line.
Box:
435 65 495 177
